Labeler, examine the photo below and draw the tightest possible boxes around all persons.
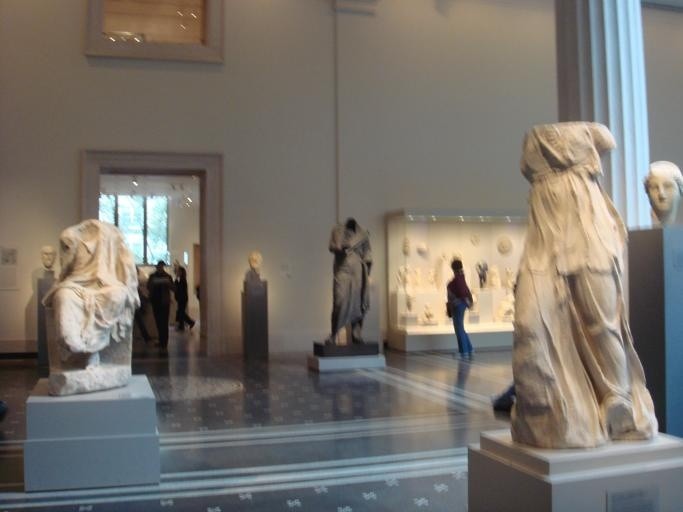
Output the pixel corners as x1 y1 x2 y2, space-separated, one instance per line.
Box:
41 219 141 360
39 244 57 269
170 266 194 332
445 259 476 356
132 264 151 344
146 259 178 358
247 250 263 270
323 216 372 344
642 161 683 229
